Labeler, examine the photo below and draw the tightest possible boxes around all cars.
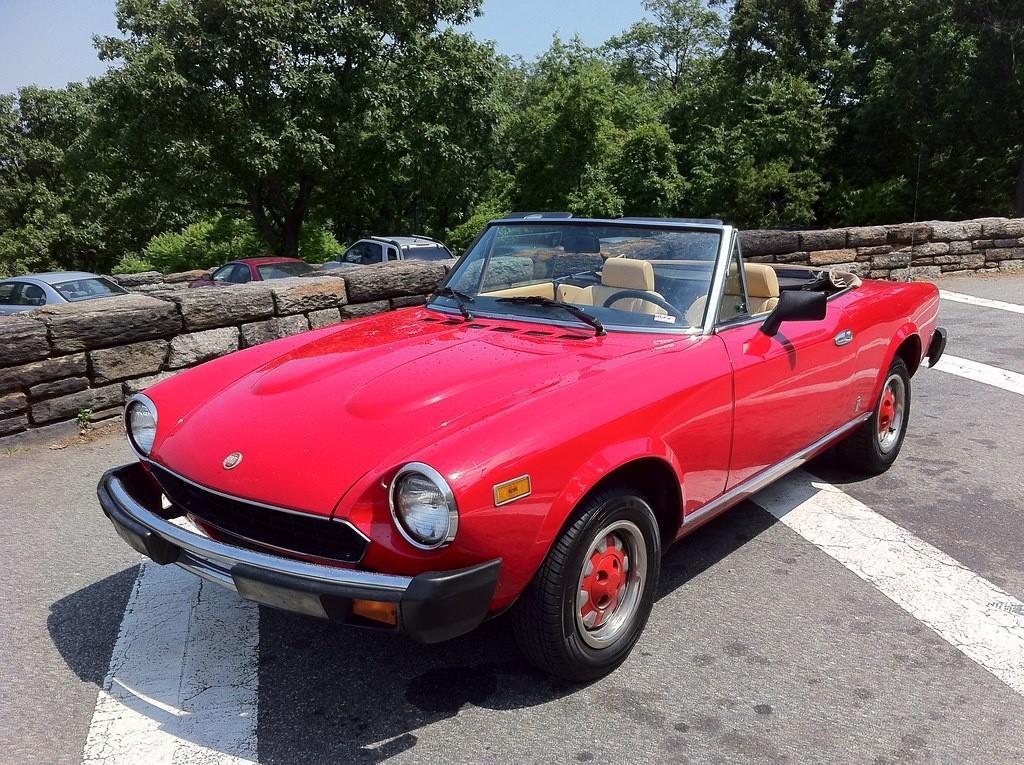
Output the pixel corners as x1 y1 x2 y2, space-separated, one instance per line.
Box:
97 211 947 686
187 255 320 288
0 271 131 316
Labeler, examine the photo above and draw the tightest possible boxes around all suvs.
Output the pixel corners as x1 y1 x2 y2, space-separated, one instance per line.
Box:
322 234 456 270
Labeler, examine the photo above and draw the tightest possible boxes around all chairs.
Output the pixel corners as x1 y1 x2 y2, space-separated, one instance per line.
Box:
21 286 42 306
685 263 781 327
63 283 77 292
570 258 668 315
